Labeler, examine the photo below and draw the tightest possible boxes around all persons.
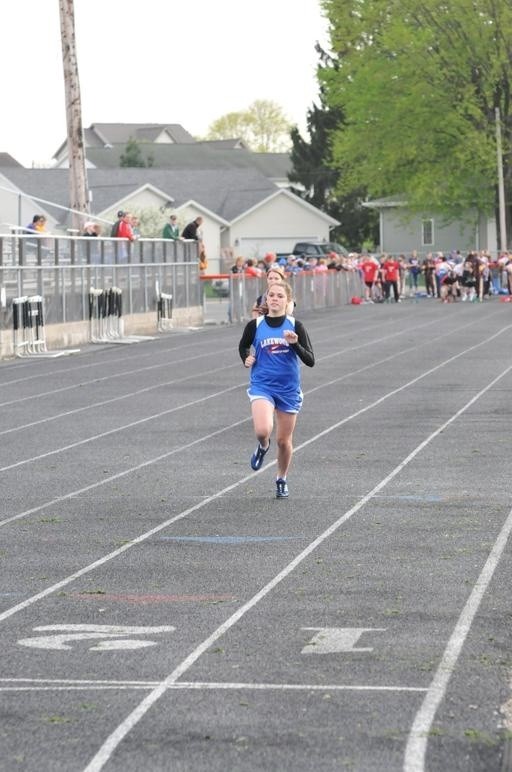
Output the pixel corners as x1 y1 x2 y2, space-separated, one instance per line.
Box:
229 250 512 320
239 282 315 498
23 211 207 274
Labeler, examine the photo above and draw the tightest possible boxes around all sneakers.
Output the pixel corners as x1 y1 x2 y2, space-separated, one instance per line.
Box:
276 479 290 499
251 439 271 471
